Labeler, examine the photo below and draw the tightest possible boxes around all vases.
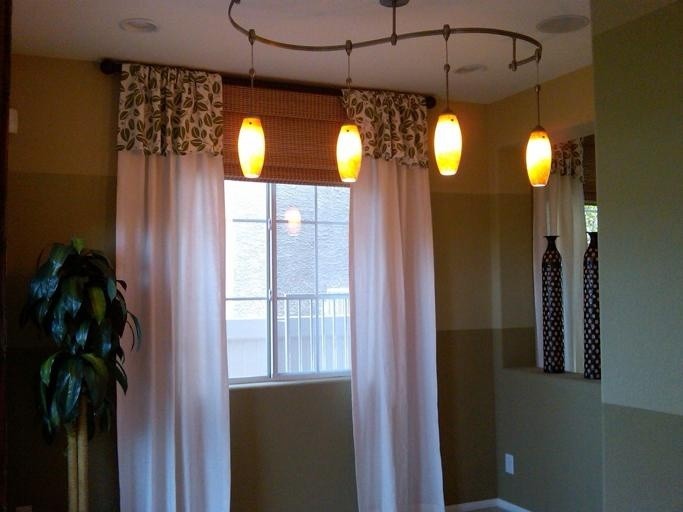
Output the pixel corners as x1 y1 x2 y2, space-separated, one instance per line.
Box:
583 231 601 380
543 234 565 372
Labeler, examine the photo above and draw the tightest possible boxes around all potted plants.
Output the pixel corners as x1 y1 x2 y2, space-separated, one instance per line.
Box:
21 239 140 512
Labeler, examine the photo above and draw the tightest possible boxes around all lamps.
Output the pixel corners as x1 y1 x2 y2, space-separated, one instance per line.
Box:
433 38 462 177
525 55 552 186
336 54 363 181
236 39 266 180
